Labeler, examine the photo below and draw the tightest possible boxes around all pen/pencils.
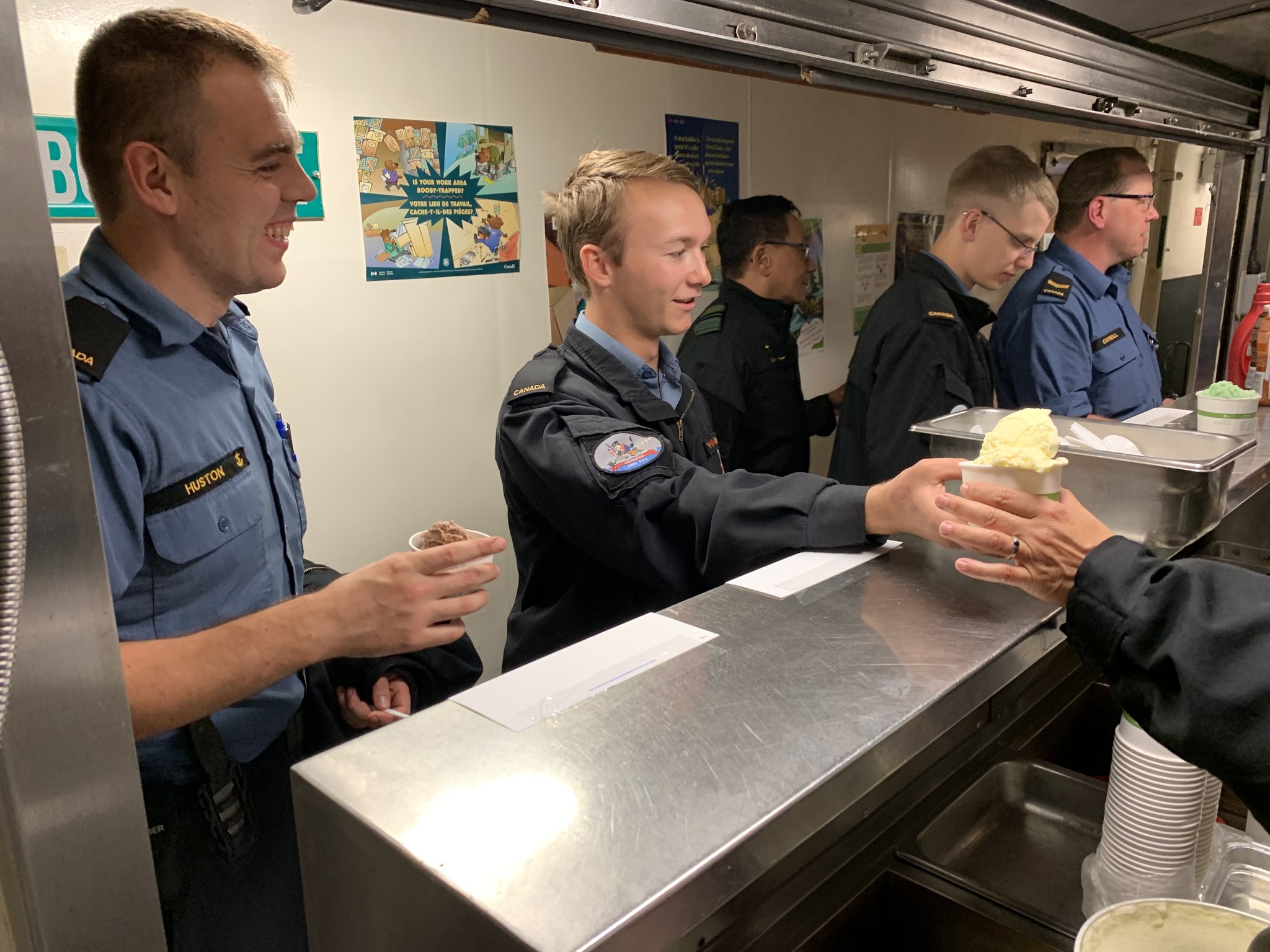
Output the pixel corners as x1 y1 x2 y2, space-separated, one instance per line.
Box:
274 411 298 460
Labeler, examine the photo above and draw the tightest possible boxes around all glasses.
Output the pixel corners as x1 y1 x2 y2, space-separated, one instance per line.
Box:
1083 192 1158 209
751 241 811 263
962 209 1038 257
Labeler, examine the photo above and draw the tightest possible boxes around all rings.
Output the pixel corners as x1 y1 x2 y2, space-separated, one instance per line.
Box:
1006 535 1019 560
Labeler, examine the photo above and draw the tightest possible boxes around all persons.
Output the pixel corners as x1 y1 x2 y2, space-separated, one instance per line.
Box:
935 484 1270 835
675 195 844 477
826 145 1058 487
989 147 1175 422
494 150 971 675
63 11 506 952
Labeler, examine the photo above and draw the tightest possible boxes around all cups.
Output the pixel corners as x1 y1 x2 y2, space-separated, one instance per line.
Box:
959 459 1068 507
408 529 494 599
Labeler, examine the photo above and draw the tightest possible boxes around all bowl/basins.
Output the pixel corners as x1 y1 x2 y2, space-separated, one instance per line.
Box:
1073 897 1269 952
1197 390 1262 437
1084 711 1222 902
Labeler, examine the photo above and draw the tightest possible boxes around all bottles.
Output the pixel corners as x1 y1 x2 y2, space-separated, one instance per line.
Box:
1227 282 1270 405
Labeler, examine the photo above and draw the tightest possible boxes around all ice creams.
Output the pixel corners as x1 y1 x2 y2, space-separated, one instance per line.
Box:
417 520 472 550
973 408 1061 472
1201 380 1258 398
